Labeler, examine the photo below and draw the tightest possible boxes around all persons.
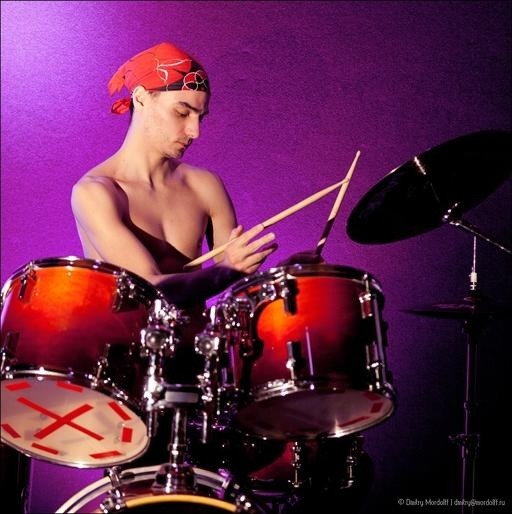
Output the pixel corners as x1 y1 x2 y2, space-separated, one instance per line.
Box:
71 43 278 354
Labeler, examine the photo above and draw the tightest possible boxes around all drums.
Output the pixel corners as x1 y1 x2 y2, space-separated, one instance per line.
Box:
0 256 191 470
216 264 395 442
52 463 269 513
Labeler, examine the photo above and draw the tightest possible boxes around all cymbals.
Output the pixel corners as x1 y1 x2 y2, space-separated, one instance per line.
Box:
396 301 512 320
346 129 511 243
